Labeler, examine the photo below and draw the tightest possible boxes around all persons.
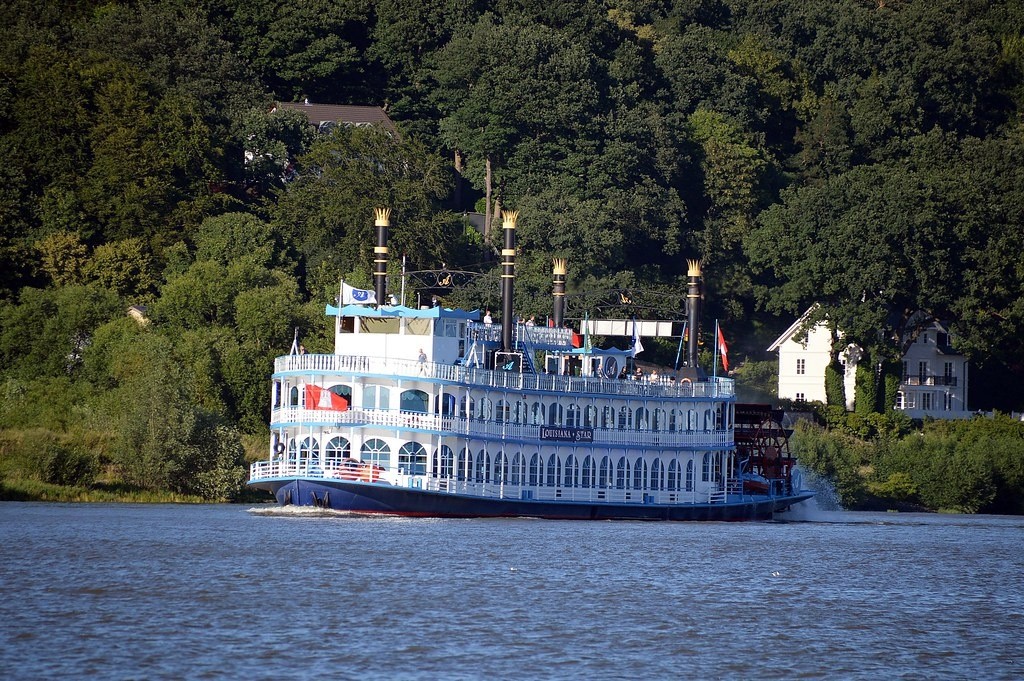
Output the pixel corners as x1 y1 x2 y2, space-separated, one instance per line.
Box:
636 366 645 396
299 344 310 353
415 349 428 377
525 314 537 327
579 364 628 394
648 369 660 396
484 310 493 329
517 317 526 323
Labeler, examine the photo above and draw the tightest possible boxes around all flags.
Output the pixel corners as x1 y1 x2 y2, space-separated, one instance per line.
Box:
717 325 728 371
342 282 378 305
305 384 348 411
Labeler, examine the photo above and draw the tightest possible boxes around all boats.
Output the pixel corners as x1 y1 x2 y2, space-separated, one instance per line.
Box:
245 203 820 518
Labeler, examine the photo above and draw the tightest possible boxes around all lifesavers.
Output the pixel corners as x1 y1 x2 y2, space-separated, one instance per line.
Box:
274 443 285 454
680 377 692 390
